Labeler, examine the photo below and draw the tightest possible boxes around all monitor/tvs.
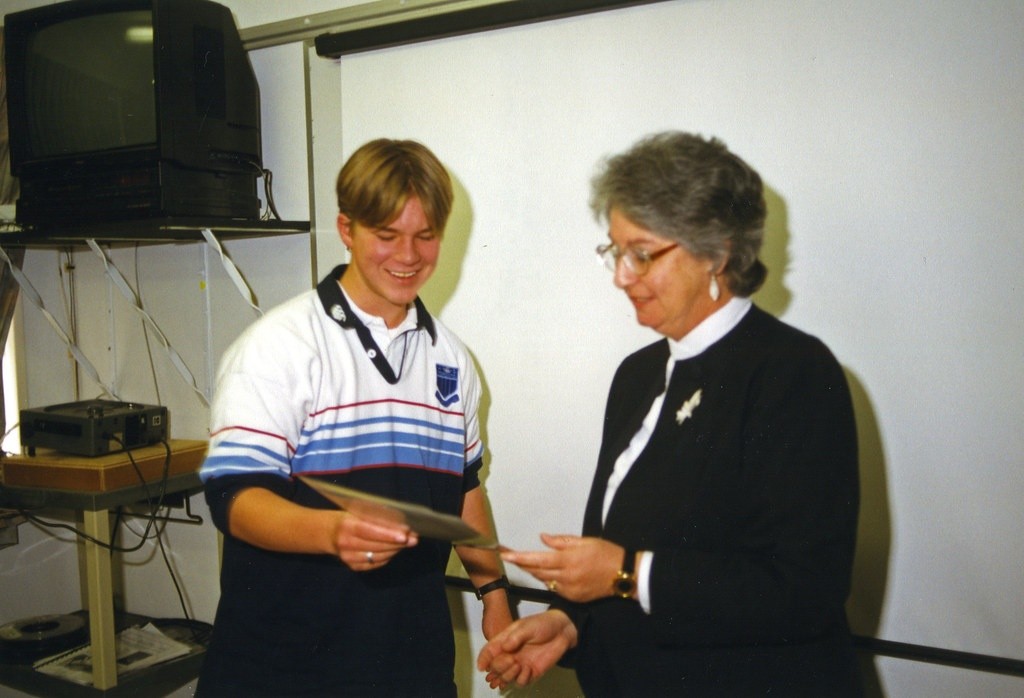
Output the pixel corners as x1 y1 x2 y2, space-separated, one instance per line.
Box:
4 0 263 178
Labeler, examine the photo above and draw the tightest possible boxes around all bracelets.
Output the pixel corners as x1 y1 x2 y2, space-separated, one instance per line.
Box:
477 575 509 600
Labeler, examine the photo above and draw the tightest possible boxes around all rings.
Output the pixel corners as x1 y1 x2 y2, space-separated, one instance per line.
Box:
366 551 374 564
549 581 558 591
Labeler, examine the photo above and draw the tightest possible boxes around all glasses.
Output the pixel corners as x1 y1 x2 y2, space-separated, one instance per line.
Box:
596 240 685 277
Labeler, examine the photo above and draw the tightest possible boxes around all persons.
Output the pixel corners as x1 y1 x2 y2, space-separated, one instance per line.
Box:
194 139 513 698
478 132 864 698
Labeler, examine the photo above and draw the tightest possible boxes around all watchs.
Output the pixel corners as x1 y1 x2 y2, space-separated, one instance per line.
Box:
614 548 636 599
